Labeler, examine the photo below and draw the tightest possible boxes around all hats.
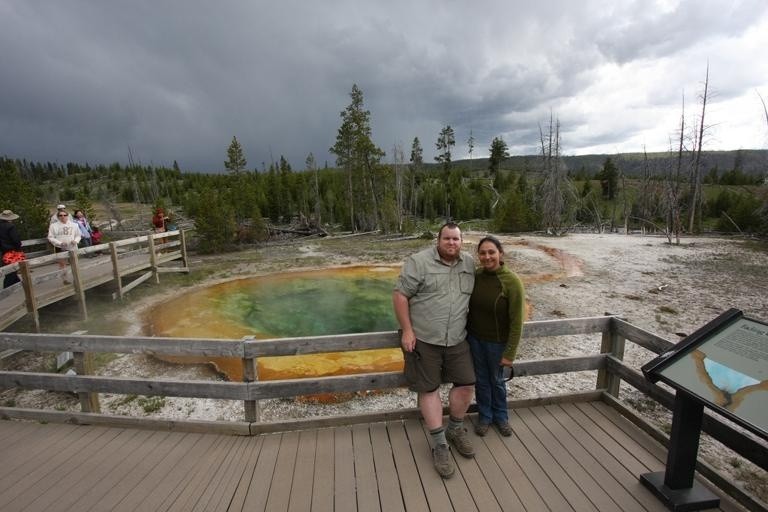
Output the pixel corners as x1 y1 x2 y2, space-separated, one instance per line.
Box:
0 210 19 221
56 205 66 209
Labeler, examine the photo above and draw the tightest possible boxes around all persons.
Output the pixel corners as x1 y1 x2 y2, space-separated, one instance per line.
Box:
392 223 479 478
90 227 103 256
152 208 171 257
48 210 81 285
0 210 22 289
465 235 525 436
73 210 96 258
50 205 74 265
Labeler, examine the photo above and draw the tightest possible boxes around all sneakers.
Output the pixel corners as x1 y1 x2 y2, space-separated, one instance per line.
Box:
475 422 489 436
445 425 476 458
430 443 455 478
497 423 512 435
